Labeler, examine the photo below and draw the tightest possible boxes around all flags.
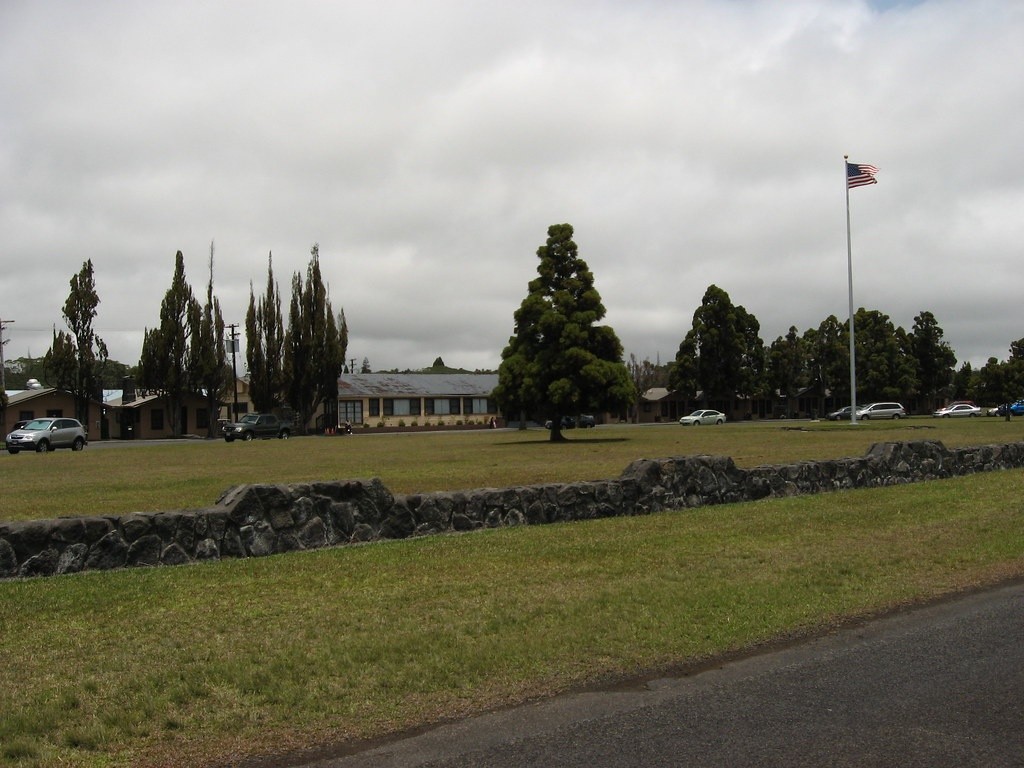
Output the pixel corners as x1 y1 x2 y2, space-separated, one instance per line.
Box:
846 161 881 190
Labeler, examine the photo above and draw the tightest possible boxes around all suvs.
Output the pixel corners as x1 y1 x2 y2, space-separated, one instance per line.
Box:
5 417 87 454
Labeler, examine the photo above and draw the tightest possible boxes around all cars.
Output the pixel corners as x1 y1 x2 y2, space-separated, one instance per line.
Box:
1006 400 1024 415
986 404 1006 417
933 404 982 419
939 401 977 412
544 413 594 429
827 405 864 421
680 409 726 426
853 402 906 420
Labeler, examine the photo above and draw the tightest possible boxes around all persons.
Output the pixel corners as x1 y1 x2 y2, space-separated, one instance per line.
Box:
344 418 352 435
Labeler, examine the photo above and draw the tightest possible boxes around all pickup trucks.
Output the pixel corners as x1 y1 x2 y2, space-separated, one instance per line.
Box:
222 413 291 443
